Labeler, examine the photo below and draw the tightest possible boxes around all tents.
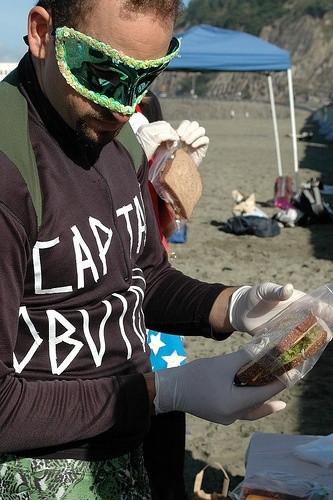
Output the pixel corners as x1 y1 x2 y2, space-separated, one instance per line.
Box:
167 24 302 201
301 104 333 144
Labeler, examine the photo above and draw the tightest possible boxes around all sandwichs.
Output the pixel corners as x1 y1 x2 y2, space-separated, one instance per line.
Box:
236 308 327 386
159 149 204 219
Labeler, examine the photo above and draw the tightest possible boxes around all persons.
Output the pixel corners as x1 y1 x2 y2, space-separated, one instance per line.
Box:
139 91 166 122
127 105 209 499
1 1 333 499
